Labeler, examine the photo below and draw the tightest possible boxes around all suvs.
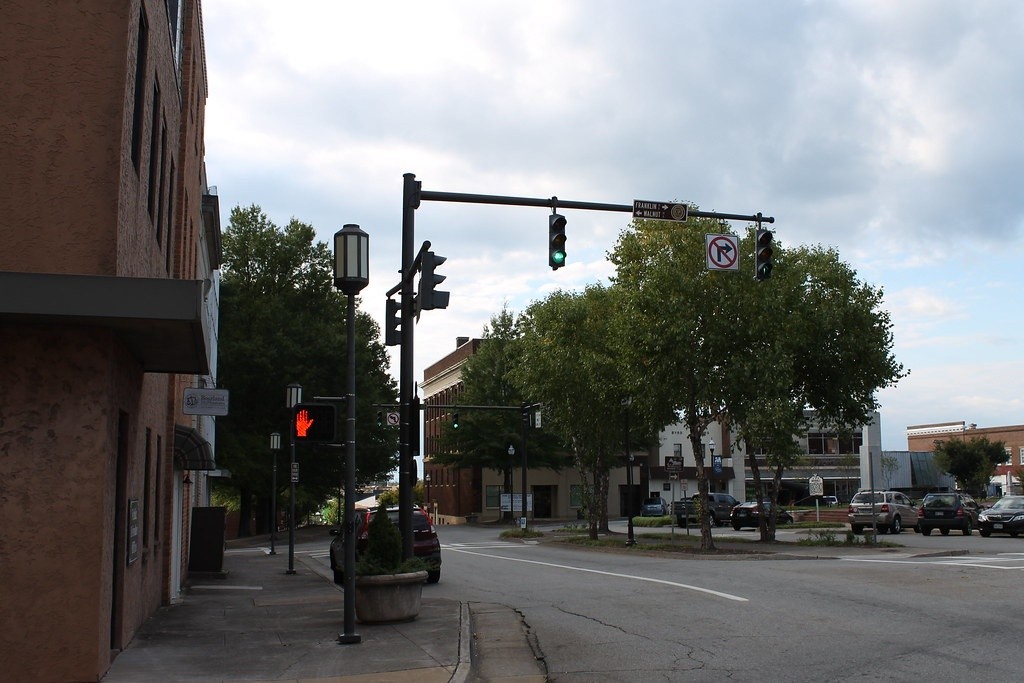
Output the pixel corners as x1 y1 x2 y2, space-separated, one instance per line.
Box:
848 489 922 535
329 504 442 587
918 491 985 537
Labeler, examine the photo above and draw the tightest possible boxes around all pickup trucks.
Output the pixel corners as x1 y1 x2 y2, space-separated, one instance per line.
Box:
671 493 741 528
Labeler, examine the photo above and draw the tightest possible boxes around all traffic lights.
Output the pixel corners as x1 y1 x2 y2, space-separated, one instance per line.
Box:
292 403 337 443
522 413 529 430
422 249 449 310
333 224 370 644
755 229 773 279
549 214 568 270
453 412 458 430
377 410 382 428
385 299 402 345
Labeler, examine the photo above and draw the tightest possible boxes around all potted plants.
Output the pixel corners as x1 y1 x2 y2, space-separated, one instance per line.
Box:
333 497 443 625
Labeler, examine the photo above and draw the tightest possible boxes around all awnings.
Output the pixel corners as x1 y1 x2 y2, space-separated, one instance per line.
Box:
174 423 216 470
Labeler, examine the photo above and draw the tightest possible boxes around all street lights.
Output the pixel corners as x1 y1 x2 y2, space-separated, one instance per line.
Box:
285 378 303 575
708 437 716 493
508 444 515 520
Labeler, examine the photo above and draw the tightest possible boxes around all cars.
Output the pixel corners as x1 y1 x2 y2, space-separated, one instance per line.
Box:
729 502 793 531
977 496 1024 537
823 496 838 505
639 497 670 516
